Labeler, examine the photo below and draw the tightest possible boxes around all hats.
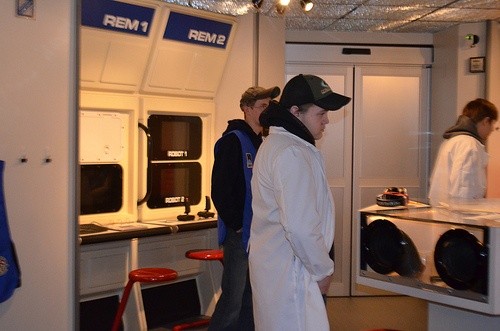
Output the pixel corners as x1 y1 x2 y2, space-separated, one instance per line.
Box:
239 86 281 111
281 74 351 111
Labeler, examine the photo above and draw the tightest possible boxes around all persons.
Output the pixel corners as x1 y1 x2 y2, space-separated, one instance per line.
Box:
249 73 353 331
211 86 280 331
427 98 499 209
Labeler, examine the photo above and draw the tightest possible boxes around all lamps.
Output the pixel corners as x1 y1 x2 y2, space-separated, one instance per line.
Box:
252 0 313 14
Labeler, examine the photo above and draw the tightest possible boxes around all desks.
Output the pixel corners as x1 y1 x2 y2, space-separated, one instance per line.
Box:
79 215 217 302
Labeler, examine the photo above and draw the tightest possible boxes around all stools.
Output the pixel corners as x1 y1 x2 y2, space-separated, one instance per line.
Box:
113 267 176 331
173 249 224 331
